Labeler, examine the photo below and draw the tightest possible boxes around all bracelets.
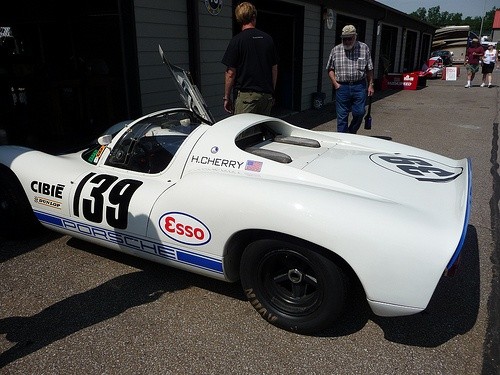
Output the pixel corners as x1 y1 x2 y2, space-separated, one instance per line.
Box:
370 83 374 85
223 98 228 101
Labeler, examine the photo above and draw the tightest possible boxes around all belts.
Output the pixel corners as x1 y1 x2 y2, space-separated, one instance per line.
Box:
239 88 256 92
338 81 359 85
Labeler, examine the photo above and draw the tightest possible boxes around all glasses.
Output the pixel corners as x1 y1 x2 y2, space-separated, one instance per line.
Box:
343 38 352 40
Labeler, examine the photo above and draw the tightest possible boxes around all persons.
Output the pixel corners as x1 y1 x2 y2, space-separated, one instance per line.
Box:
480 43 498 88
220 2 278 116
326 24 375 133
465 39 484 87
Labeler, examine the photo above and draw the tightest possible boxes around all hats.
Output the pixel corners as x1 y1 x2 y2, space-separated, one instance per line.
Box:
342 25 356 36
470 38 480 44
487 43 495 46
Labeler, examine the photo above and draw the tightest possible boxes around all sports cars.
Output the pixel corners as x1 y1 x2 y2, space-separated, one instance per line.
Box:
0 44 471 335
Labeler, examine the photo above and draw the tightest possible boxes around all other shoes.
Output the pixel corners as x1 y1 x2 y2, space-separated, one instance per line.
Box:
471 81 472 87
488 83 492 88
480 83 485 87
465 80 470 87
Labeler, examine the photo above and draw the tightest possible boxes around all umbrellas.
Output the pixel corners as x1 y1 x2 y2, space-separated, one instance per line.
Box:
421 57 439 71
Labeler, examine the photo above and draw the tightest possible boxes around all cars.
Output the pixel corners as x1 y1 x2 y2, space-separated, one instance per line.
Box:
431 50 454 67
